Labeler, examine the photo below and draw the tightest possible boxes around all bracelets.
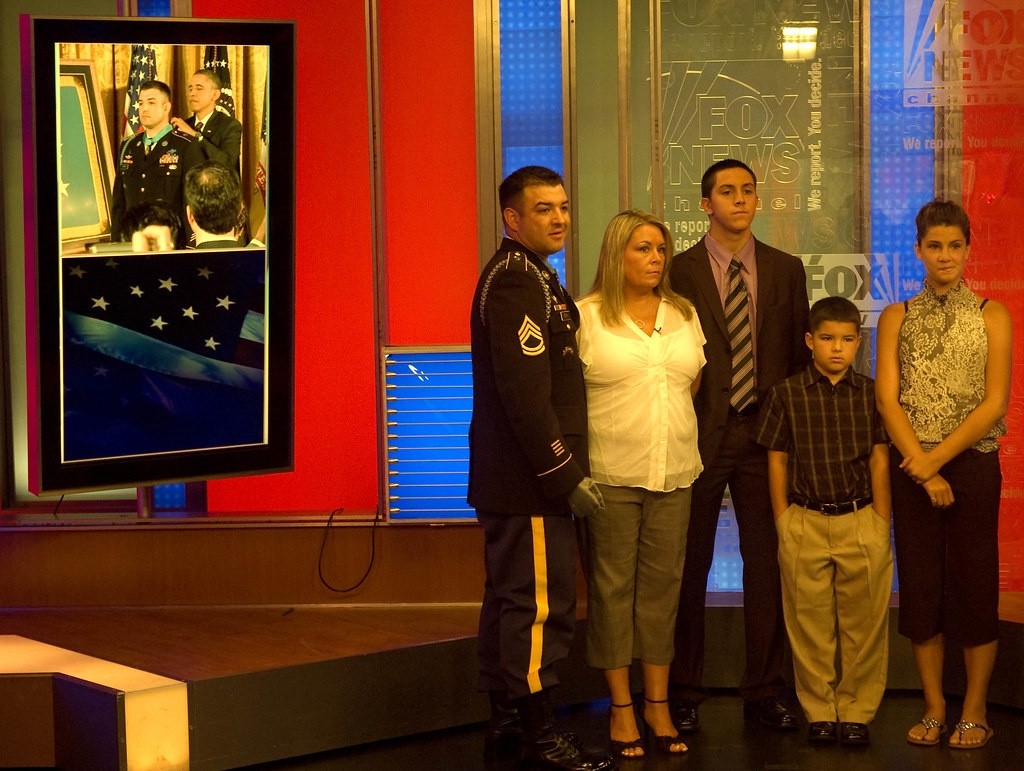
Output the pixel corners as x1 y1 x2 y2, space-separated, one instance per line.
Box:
195 130 201 137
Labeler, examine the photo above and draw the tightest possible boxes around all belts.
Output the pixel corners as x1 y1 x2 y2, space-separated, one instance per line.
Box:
790 493 873 516
729 403 756 416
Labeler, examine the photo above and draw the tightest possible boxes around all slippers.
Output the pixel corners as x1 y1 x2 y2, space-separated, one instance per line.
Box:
906 717 948 745
948 719 994 749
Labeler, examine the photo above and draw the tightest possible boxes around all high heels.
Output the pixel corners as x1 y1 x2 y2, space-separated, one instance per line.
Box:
606 701 647 761
639 695 690 757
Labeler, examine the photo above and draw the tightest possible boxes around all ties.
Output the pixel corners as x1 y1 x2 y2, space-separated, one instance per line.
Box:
194 122 203 132
553 272 565 295
725 254 755 413
145 138 154 155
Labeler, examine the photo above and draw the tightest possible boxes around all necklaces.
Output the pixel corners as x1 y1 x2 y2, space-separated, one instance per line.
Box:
623 299 654 328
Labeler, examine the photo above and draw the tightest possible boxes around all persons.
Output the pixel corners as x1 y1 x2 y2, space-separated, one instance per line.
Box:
467 165 612 770
184 159 244 248
111 81 206 248
169 69 242 171
874 195 1012 748
667 158 813 729
570 209 708 760
754 296 890 747
120 201 181 250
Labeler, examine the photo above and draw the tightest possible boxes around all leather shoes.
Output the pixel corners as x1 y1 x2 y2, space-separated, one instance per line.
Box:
483 704 615 771
840 722 870 747
742 698 802 732
668 698 702 731
808 722 839 746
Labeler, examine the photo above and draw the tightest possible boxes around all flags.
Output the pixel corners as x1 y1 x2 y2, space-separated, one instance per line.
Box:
119 43 159 139
205 45 234 117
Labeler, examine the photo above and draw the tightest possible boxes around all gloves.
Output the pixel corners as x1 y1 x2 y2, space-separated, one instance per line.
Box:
565 477 606 518
